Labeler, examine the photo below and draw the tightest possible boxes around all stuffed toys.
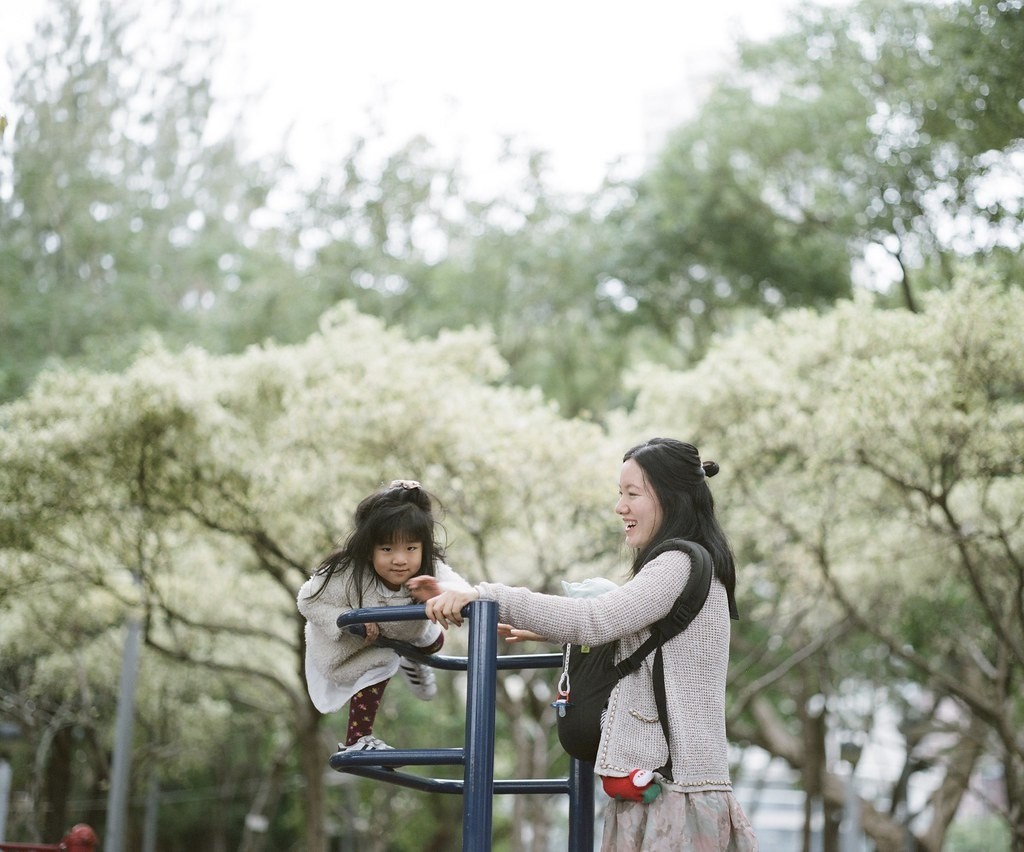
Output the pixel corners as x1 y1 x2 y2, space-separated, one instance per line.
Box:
598 768 662 803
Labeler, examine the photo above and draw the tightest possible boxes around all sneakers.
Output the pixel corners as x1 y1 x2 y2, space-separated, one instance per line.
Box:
399 654 438 702
337 735 395 751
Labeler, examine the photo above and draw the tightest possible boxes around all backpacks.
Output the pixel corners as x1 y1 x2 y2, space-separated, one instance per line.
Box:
550 537 713 763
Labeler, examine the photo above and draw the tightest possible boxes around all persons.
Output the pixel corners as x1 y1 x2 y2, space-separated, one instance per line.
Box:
296 479 475 753
421 437 762 851
